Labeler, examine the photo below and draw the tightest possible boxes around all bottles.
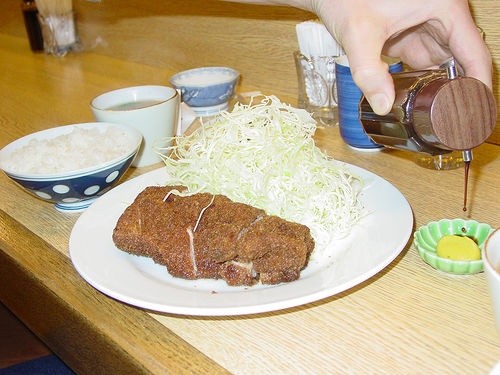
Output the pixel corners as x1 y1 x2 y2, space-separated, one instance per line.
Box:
20 0 43 53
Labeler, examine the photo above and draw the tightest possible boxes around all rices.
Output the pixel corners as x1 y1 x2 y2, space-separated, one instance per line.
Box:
4 126 133 174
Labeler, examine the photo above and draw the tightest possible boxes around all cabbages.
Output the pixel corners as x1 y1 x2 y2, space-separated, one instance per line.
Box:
153 95 370 249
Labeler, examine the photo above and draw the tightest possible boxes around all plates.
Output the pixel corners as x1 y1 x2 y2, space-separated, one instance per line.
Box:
413 218 496 274
68 159 415 318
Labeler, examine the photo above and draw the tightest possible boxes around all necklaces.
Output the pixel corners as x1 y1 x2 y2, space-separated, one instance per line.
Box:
476 26 484 39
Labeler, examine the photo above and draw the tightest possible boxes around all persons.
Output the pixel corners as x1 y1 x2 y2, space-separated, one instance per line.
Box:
237 0 492 115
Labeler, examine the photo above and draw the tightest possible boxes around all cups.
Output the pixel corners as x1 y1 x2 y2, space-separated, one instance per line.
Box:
482 226 500 325
358 58 500 164
37 10 87 58
335 54 403 153
88 85 180 168
294 50 345 129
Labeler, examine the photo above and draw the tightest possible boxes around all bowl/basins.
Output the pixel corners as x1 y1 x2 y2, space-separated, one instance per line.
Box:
172 66 241 117
0 121 144 214
